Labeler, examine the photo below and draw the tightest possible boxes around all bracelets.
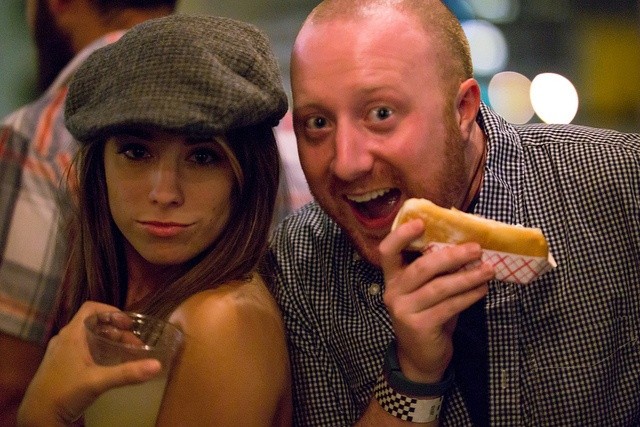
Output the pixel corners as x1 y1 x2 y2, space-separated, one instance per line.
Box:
383 339 456 396
375 379 444 423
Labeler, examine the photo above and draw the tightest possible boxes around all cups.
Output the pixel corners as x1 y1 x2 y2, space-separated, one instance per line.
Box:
84 312 185 426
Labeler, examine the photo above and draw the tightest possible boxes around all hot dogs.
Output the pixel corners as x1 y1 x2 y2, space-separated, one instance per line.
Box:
392 197 558 285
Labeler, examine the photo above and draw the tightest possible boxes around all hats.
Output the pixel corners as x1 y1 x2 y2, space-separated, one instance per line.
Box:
64 14 288 141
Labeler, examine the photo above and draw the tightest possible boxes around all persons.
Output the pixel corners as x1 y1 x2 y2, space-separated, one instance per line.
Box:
1 0 178 425
14 13 295 427
29 2 79 102
268 0 639 427
